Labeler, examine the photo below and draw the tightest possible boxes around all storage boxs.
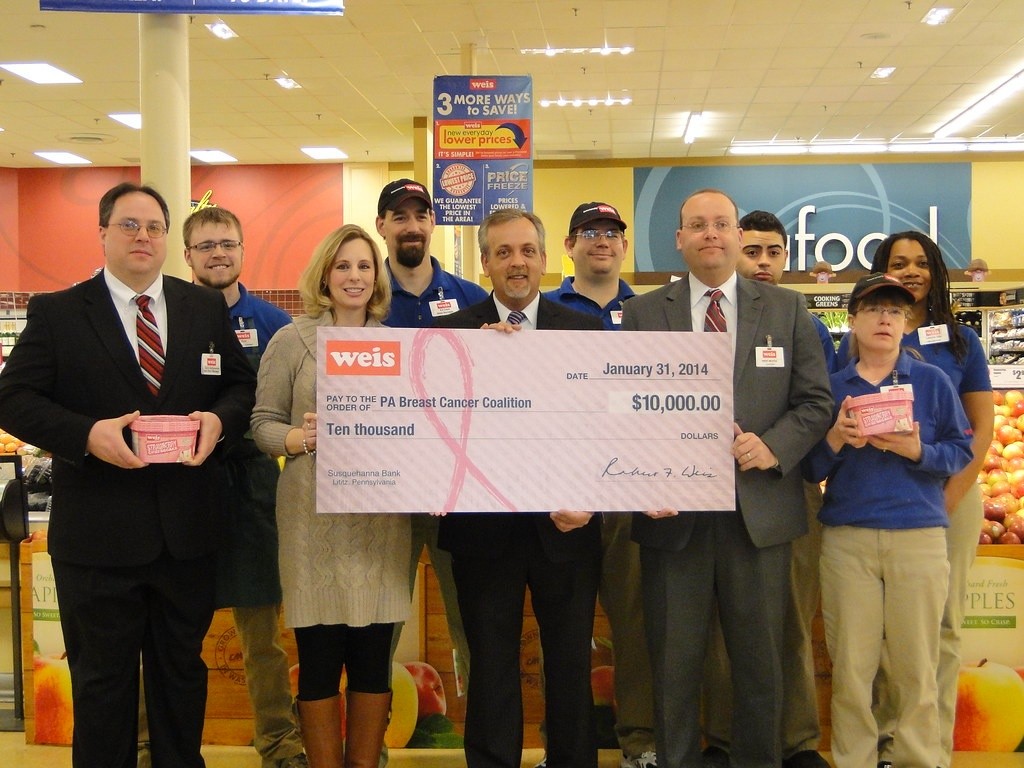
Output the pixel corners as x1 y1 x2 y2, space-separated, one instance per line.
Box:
846 391 913 437
128 414 201 463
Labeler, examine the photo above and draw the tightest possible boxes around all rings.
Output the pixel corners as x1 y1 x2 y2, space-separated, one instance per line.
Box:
747 453 752 461
308 422 310 429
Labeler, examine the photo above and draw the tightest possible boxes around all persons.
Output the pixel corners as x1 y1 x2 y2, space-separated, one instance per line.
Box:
0 179 309 768
250 180 835 768
804 274 972 767
839 230 994 768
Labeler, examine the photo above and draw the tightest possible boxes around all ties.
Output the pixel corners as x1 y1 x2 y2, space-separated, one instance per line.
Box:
507 311 528 329
130 295 167 394
703 288 728 333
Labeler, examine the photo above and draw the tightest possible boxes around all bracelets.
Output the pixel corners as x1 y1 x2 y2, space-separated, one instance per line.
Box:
302 437 316 456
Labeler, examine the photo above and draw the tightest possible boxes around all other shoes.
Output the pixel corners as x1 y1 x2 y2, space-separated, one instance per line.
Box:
532 758 547 767
700 747 730 768
621 750 658 768
782 750 830 768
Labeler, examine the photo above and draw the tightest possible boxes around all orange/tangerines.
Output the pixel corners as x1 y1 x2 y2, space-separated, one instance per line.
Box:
385 661 419 749
0 429 24 453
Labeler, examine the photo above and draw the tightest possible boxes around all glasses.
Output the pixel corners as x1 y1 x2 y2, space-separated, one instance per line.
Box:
853 304 907 318
679 220 737 233
573 229 624 241
188 241 243 250
105 220 168 239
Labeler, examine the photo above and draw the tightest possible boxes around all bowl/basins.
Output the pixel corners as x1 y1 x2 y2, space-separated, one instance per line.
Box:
847 391 914 439
128 415 201 463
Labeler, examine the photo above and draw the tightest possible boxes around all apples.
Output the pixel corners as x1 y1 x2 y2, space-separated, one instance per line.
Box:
977 391 1024 544
289 663 347 740
34 652 74 746
953 658 1024 752
592 666 615 706
403 661 447 721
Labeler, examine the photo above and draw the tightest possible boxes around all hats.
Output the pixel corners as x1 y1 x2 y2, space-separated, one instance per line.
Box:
377 178 438 210
849 272 917 307
566 200 630 231
